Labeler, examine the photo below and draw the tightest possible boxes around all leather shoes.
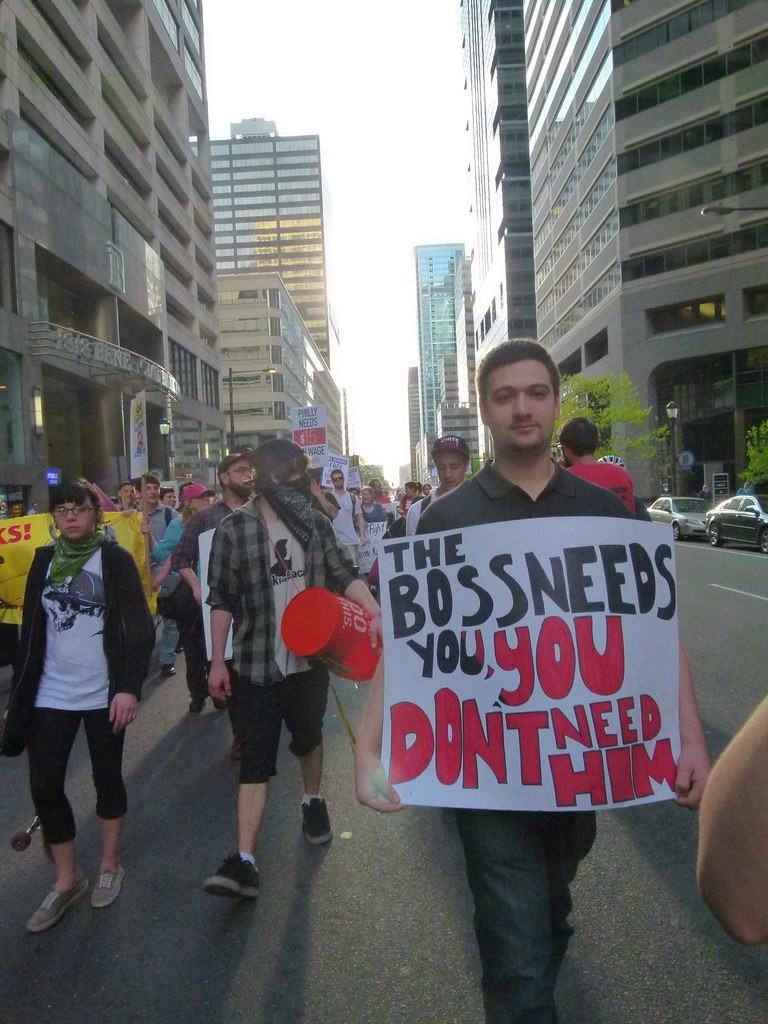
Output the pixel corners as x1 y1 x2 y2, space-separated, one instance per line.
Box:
160 663 176 676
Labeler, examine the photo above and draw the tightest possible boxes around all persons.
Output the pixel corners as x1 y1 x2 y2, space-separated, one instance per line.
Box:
355 338 709 1024
299 466 433 579
558 417 651 522
169 450 256 759
736 482 754 496
199 436 383 903
0 474 154 933
0 470 217 715
406 435 470 536
698 696 768 945
698 484 713 503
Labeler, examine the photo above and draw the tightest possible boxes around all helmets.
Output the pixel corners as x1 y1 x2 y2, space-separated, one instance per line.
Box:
598 454 627 473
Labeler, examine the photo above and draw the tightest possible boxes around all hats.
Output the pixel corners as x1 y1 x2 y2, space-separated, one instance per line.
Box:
218 452 254 473
160 485 175 499
430 435 471 458
181 483 216 500
118 480 134 491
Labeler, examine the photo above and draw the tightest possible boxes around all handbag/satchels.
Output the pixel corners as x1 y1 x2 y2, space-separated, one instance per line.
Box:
0 701 32 757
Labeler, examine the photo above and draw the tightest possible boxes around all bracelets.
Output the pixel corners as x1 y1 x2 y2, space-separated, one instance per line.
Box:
367 613 382 621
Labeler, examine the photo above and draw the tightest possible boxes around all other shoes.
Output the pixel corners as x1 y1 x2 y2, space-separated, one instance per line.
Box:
175 643 184 653
211 697 228 710
188 696 205 712
229 735 242 760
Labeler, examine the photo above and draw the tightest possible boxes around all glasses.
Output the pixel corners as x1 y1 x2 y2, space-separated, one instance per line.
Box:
555 442 565 450
224 466 253 474
333 476 343 481
374 484 383 488
50 504 98 517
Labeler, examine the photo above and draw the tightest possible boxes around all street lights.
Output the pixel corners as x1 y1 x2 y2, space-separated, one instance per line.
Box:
228 367 277 453
159 417 171 481
550 443 558 458
666 402 678 496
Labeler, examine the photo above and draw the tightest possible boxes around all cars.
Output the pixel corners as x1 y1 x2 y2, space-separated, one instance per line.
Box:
647 496 713 541
705 494 768 554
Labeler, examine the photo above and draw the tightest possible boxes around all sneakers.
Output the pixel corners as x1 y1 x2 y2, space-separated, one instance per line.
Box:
90 862 125 908
25 869 90 932
302 797 333 845
201 853 260 898
